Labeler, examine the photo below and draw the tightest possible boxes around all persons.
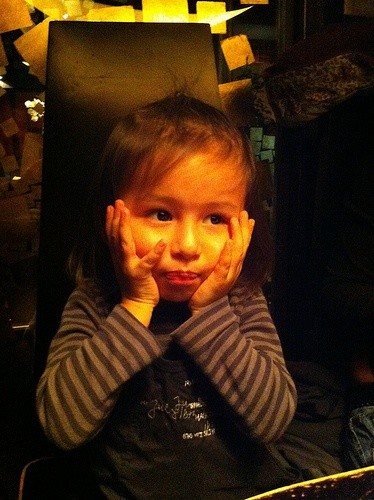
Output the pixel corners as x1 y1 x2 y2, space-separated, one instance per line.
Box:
36 93 297 500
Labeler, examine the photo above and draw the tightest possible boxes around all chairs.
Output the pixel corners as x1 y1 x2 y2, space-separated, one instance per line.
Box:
16 22 222 500
